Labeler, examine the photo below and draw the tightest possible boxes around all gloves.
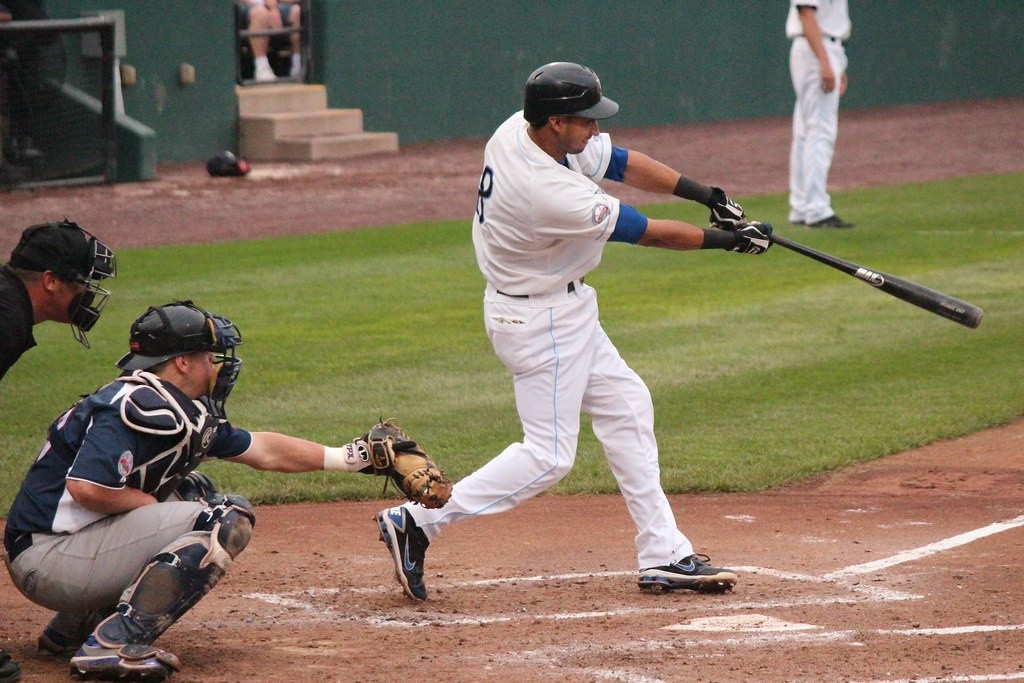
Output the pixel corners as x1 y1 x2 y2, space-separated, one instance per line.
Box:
707 186 774 255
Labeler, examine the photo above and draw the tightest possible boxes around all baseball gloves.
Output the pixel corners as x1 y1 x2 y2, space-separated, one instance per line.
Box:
356 416 455 509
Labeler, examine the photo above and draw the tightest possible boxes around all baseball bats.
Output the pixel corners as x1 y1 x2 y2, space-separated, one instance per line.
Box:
772 235 984 330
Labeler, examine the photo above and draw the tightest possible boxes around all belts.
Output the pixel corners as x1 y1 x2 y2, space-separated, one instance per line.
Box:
497 277 585 299
831 37 844 46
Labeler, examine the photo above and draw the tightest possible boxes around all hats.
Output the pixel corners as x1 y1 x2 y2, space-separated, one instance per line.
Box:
9 223 114 280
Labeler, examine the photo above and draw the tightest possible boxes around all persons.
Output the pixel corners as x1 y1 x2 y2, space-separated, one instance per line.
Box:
0 0 67 185
239 0 301 83
372 61 772 602
0 222 116 683
786 0 855 228
4 302 452 679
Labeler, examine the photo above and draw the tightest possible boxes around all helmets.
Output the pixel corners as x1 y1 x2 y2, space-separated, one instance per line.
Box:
524 62 619 125
115 304 234 371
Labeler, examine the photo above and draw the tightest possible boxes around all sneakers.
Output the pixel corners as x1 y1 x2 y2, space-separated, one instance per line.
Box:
636 553 737 594
39 625 79 653
792 215 854 229
372 506 429 602
69 635 173 682
